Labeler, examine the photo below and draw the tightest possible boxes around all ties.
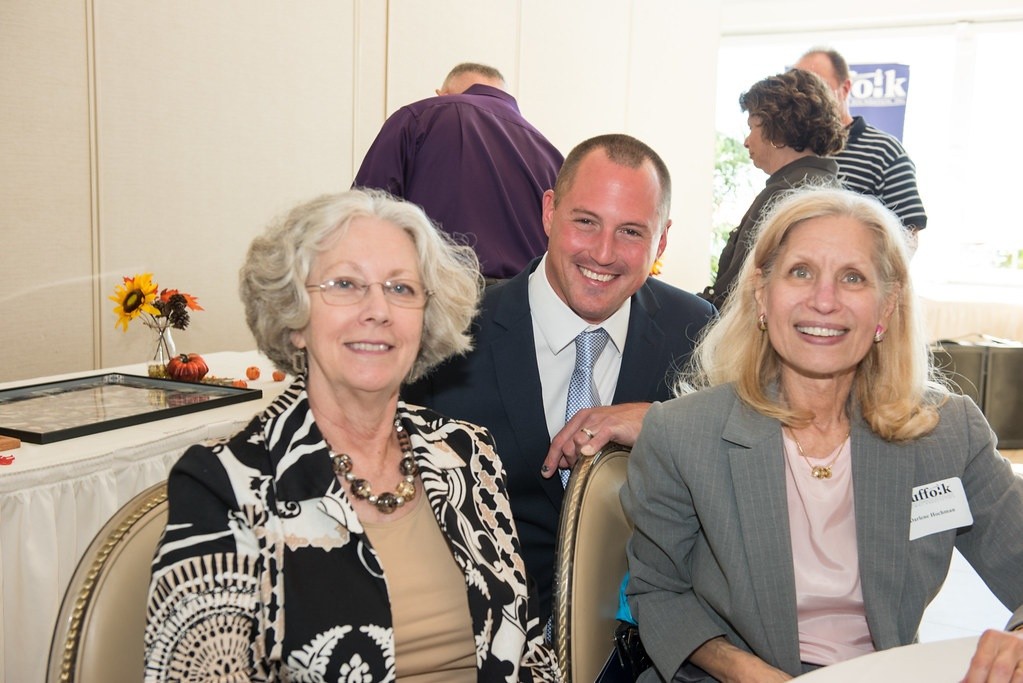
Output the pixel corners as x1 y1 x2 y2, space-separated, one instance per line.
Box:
544 328 610 646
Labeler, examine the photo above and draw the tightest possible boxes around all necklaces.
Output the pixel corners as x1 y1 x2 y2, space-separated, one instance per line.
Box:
322 412 419 514
788 424 850 479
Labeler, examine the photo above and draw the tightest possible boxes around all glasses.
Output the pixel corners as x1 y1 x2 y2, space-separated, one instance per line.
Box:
305 276 435 308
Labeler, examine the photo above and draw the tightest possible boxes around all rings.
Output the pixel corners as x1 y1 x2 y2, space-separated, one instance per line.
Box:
580 427 594 440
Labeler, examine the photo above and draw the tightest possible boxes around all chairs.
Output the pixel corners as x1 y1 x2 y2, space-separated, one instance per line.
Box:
44 479 170 683
550 439 637 683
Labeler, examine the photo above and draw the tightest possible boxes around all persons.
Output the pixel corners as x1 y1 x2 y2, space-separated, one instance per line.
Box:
790 47 928 257
142 188 563 683
353 63 566 289
400 134 720 629
625 173 1023 682
695 66 847 310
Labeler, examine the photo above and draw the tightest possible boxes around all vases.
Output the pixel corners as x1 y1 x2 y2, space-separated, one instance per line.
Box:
148 322 180 380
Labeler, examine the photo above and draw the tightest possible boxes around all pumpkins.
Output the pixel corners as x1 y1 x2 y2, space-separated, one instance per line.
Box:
231 366 285 388
167 352 209 383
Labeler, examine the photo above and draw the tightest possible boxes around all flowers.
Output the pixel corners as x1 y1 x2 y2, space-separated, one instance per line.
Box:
108 272 206 361
